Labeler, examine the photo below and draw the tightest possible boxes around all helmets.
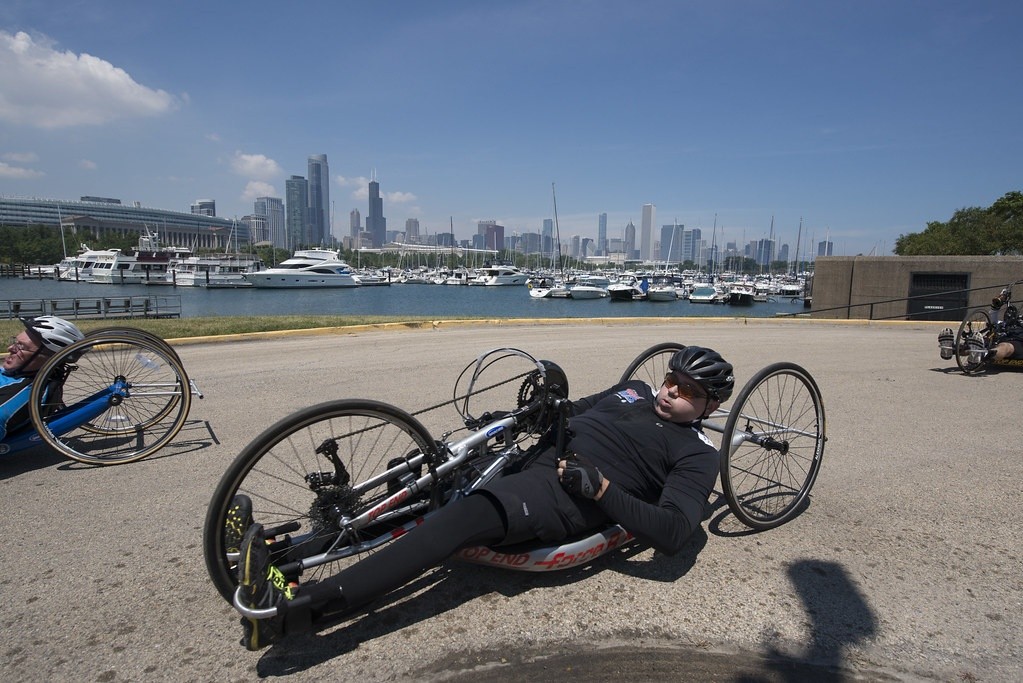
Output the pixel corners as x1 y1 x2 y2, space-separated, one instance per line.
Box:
20 315 86 362
668 346 735 403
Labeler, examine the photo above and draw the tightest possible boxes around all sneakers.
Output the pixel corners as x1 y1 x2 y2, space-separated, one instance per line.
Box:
223 494 254 582
238 523 297 652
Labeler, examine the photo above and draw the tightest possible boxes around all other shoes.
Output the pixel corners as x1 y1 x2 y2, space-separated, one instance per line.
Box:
969 332 984 367
938 328 954 360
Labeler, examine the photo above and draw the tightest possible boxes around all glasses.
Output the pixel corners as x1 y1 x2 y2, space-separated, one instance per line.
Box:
664 372 709 399
8 336 47 358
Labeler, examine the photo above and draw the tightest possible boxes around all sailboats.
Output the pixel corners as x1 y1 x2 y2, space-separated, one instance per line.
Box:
25 181 814 306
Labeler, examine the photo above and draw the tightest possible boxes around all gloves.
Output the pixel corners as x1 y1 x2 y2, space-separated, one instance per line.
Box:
991 293 1007 311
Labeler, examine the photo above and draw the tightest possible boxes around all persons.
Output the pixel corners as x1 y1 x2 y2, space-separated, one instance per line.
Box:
0 315 89 442
938 287 1023 367
225 346 736 652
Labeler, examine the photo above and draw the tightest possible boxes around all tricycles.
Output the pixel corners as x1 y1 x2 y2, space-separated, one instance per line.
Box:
0 326 203 467
201 341 828 621
937 279 1023 376
